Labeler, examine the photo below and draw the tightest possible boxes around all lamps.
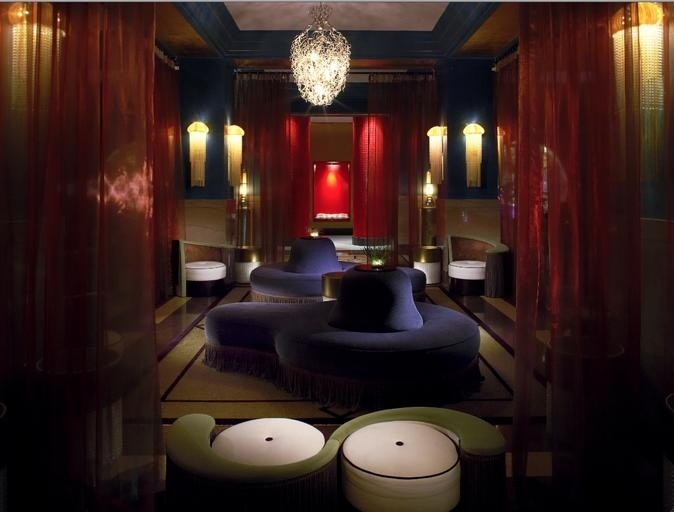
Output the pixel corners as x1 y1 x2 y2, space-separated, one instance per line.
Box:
422 170 437 207
424 124 446 187
221 124 246 190
238 171 251 208
185 116 213 189
287 3 354 108
461 123 486 189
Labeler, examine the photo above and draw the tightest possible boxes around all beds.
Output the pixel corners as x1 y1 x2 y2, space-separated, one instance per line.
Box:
313 212 351 228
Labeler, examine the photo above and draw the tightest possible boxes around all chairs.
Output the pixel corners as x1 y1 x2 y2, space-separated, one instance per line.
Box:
203 269 486 405
248 236 428 304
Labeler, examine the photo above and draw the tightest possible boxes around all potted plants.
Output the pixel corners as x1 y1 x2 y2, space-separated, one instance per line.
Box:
363 244 392 268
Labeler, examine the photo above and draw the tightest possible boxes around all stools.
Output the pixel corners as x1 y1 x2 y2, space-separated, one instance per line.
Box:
233 245 265 287
322 272 345 302
187 259 228 298
414 246 441 284
341 420 463 511
447 258 485 298
211 417 325 467
